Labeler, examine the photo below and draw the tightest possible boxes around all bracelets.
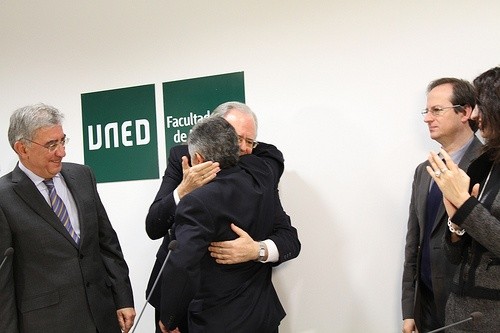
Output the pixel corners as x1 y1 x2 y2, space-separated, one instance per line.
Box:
447 217 465 236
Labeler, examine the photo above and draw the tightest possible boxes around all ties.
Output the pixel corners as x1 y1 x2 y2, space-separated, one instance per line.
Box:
420 158 446 288
43 178 79 245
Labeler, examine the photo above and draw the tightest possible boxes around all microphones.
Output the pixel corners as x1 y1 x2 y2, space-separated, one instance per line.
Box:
132 240 177 333
0 247 14 269
428 312 484 333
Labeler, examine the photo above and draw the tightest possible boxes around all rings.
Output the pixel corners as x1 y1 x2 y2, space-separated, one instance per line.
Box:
434 170 441 178
201 175 205 179
441 167 448 174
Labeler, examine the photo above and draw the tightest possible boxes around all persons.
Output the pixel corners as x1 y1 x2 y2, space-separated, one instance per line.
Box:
0 104 137 333
145 102 301 333
401 77 484 333
426 67 500 333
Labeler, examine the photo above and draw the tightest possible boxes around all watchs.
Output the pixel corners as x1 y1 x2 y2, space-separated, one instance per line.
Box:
256 241 265 262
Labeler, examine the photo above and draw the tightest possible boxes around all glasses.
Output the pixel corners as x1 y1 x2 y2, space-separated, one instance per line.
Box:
420 104 464 118
19 137 69 152
237 136 259 149
